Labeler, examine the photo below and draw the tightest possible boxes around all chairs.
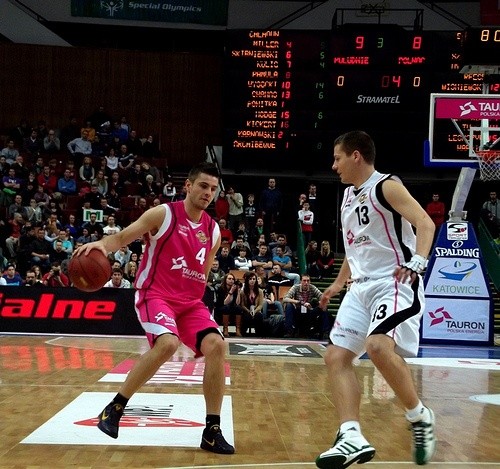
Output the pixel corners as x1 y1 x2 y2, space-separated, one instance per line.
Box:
229 269 292 300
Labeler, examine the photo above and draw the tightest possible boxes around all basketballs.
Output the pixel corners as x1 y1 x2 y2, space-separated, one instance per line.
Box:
68 248 112 292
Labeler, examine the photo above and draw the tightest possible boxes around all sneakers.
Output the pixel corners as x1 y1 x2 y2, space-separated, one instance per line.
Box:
404 408 438 466
316 428 377 469
97 401 123 440
200 425 235 455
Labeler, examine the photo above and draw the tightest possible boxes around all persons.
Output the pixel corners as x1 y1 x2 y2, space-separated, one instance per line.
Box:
0 104 336 339
315 130 438 469
72 161 234 454
482 191 500 242
426 193 446 254
